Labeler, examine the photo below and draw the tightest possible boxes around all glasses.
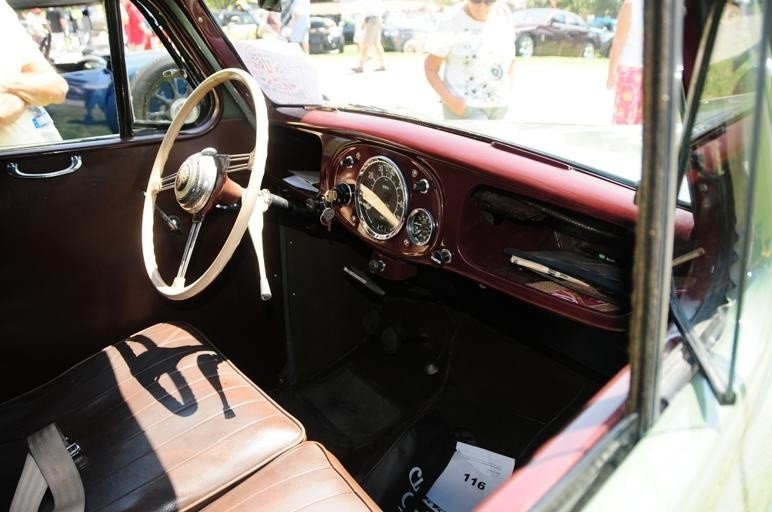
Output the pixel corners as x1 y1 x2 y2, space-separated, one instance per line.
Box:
470 0 497 6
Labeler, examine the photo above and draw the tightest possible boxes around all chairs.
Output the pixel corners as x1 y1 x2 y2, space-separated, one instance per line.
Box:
2 314 382 512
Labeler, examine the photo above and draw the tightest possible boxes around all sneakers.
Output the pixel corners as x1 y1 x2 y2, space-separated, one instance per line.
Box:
352 66 387 73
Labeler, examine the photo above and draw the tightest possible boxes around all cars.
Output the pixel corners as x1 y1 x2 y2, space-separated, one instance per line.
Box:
42 28 187 132
508 6 616 59
308 3 426 55
